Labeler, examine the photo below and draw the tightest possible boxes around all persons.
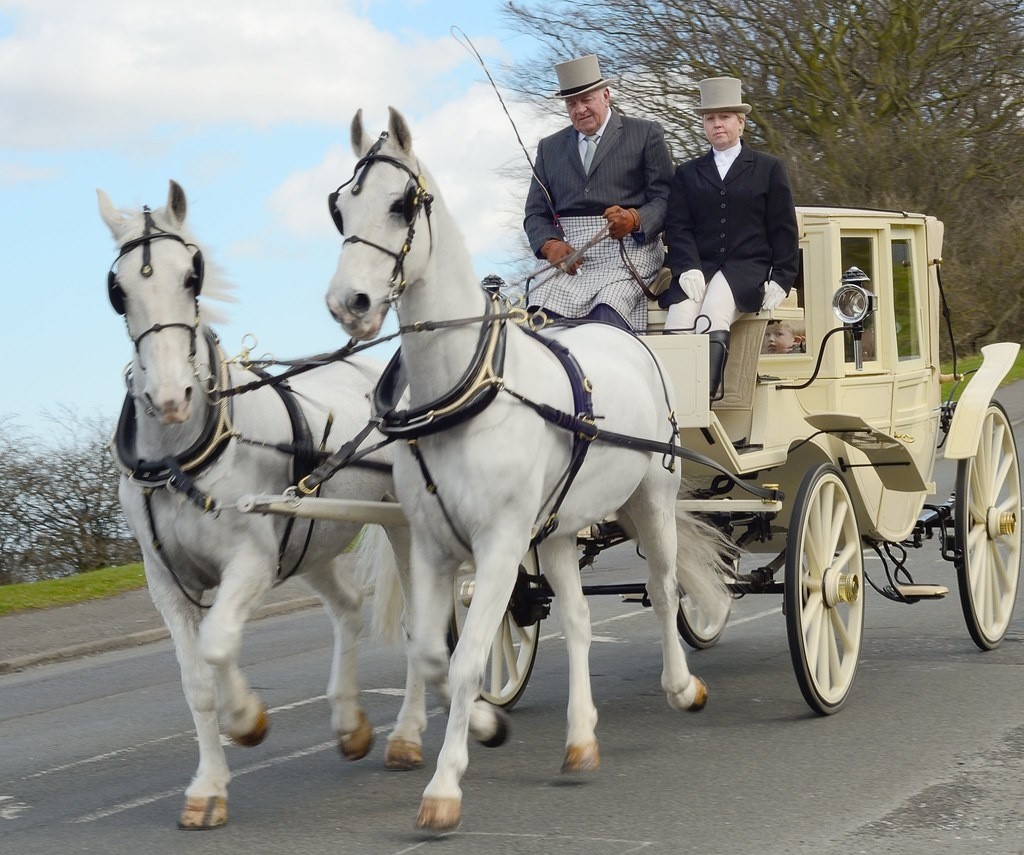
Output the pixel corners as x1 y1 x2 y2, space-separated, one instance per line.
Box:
660 75 799 414
522 52 676 337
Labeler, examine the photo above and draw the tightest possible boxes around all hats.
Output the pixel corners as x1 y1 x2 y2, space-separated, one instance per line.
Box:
691 78 753 115
553 54 611 98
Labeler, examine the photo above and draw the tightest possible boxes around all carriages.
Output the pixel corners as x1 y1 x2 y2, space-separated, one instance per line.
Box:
91 109 1023 834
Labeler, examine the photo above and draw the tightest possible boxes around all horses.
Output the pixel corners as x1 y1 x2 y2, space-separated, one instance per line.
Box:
324 106 751 831
98 179 429 830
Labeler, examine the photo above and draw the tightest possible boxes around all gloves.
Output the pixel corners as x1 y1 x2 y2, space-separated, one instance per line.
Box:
761 281 786 310
603 205 640 240
543 240 582 277
678 269 705 301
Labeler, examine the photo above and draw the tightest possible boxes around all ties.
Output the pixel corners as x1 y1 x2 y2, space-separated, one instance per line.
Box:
584 135 599 177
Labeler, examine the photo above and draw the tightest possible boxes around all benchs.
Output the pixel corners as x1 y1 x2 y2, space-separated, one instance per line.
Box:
647 270 807 410
